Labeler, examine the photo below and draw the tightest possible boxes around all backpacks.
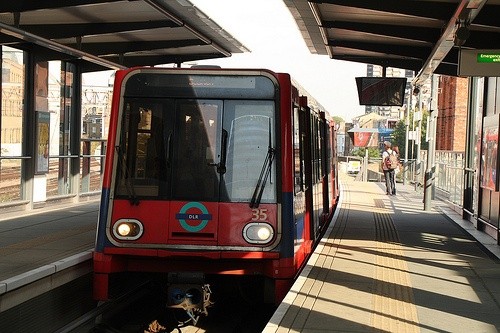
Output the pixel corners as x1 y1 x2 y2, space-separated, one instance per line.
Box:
385 150 398 169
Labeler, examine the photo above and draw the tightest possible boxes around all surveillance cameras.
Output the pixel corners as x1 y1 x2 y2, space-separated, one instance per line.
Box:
453 26 470 46
413 88 419 96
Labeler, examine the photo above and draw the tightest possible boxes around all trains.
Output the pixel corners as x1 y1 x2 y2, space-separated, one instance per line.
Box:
90 64 340 327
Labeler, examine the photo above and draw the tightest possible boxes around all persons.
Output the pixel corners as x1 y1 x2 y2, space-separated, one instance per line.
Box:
381 141 397 195
387 146 400 189
